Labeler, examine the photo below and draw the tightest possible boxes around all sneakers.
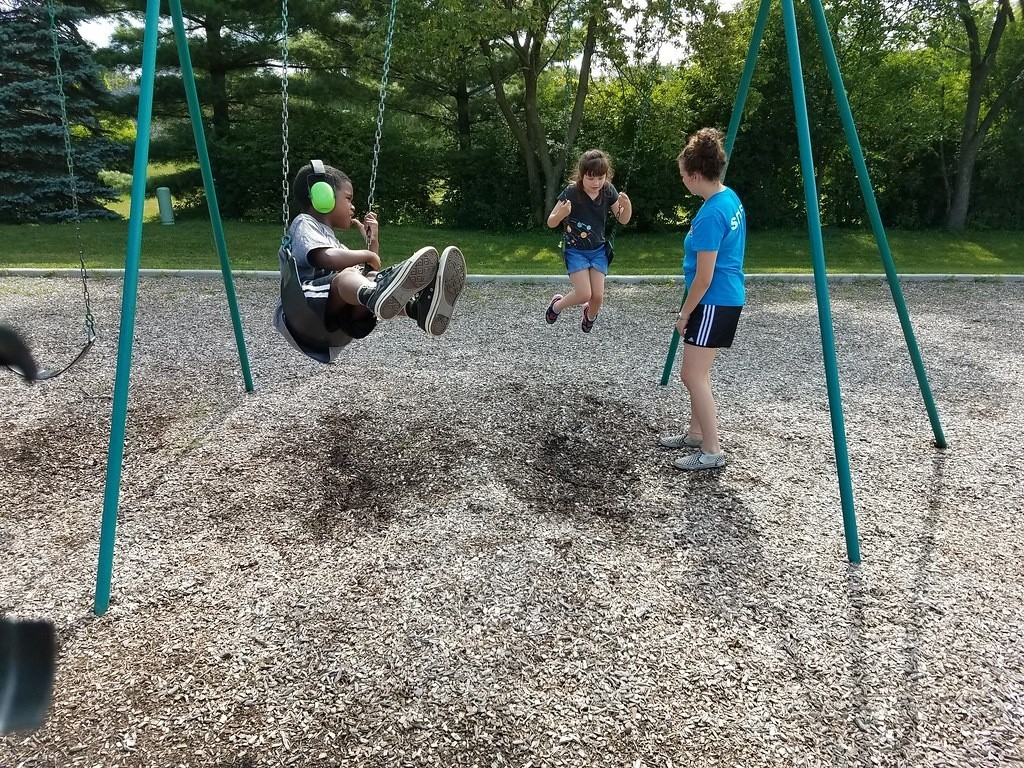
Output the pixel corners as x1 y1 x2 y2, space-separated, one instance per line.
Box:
545 294 562 324
580 302 597 333
415 246 467 336
367 246 439 320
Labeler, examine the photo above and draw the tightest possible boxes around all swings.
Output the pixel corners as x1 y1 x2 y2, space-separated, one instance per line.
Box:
277 0 399 353
557 0 675 279
0 0 100 382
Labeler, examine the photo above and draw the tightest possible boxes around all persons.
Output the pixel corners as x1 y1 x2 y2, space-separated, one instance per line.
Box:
545 149 633 333
277 163 467 336
659 127 746 471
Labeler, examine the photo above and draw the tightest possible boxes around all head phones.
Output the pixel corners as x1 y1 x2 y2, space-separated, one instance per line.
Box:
307 159 336 214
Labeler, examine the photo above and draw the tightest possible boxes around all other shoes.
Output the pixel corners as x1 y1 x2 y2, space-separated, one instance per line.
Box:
659 436 703 449
675 449 725 469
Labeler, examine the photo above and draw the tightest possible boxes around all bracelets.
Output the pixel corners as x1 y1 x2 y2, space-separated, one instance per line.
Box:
678 312 690 321
